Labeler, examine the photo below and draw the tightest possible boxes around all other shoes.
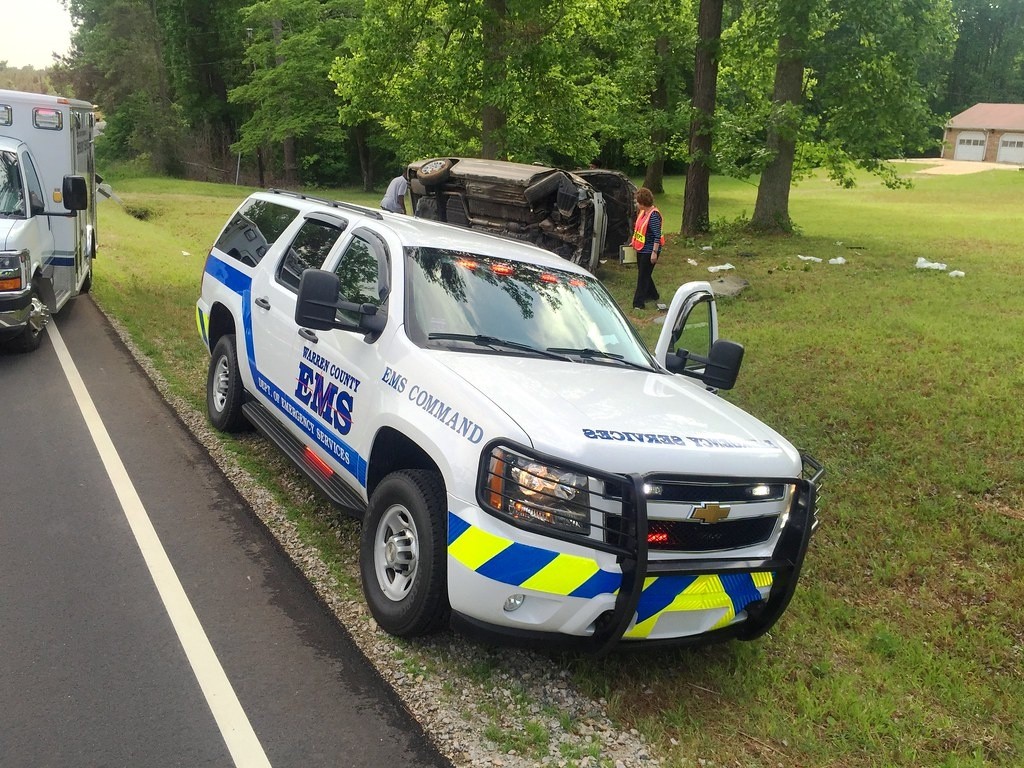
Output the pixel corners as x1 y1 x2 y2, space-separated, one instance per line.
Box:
646 297 654 303
635 304 645 309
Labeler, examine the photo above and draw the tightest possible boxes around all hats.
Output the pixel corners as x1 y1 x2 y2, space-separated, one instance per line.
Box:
589 158 602 166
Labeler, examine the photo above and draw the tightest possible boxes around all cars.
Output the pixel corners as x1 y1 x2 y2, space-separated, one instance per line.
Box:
406 156 638 279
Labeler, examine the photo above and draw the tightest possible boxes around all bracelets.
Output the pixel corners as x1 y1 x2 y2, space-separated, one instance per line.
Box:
652 249 658 253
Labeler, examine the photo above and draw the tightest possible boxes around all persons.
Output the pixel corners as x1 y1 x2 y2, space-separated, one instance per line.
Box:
627 188 665 314
379 170 409 215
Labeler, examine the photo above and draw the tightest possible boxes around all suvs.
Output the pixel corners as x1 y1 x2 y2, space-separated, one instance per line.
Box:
193 189 825 646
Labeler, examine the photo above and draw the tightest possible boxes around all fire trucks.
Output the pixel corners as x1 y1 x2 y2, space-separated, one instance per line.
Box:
0 90 100 355
